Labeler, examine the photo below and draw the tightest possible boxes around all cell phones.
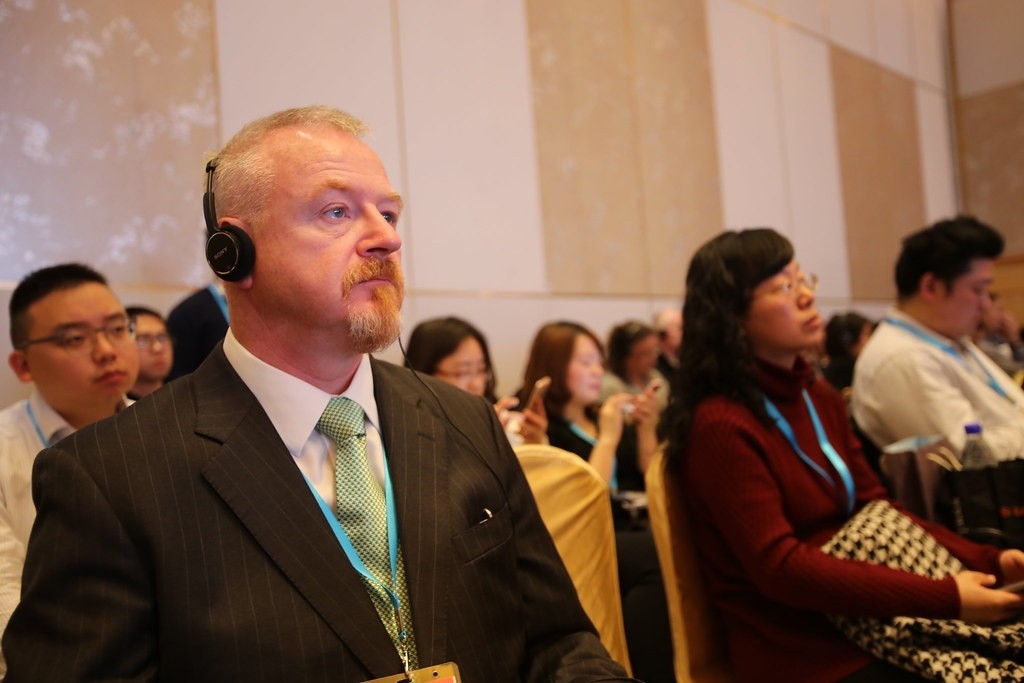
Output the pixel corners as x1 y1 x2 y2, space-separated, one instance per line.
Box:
998 581 1024 594
516 375 550 435
626 379 664 424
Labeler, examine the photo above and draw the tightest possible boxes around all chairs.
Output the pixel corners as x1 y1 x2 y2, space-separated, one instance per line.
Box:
642 444 718 683
511 444 633 683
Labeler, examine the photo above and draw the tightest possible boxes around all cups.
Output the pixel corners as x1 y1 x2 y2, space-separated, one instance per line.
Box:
588 369 639 424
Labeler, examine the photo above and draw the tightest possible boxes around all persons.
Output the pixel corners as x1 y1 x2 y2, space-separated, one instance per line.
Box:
1 263 142 680
126 307 173 401
0 105 634 683
825 312 871 389
974 292 1024 378
164 275 229 382
853 215 1024 517
656 228 1024 683
403 308 683 683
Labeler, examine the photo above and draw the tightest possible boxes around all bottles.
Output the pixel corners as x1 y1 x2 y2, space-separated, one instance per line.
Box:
960 423 997 469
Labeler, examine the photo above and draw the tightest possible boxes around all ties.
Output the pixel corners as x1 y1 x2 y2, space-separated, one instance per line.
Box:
316 398 419 672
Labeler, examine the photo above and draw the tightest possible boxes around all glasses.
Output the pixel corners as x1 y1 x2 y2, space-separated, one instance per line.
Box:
435 365 492 385
18 317 137 357
752 274 816 301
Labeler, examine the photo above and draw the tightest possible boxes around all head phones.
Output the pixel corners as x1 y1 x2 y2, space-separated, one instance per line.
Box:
203 158 256 282
